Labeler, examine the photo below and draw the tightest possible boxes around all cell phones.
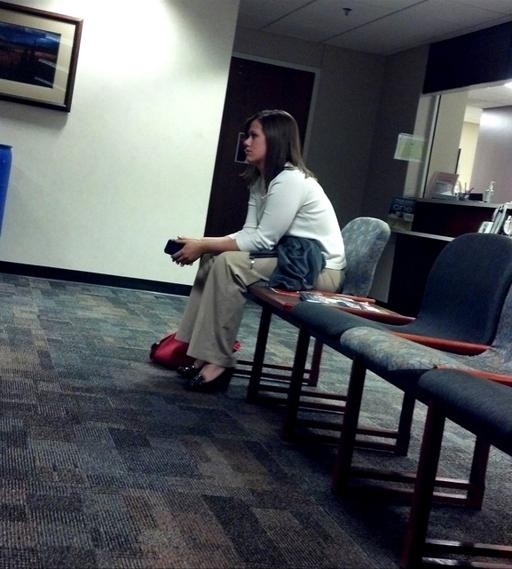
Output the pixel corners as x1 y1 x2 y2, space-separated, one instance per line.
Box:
164 239 185 255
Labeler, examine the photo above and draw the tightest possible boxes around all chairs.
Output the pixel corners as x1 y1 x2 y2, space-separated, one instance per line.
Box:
278 216 511 569
235 217 396 387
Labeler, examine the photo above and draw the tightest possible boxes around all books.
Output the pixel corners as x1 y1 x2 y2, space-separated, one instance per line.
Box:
298 291 382 313
385 194 418 233
477 200 512 238
428 190 462 202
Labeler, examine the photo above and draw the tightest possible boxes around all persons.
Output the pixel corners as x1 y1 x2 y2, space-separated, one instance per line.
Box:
169 108 348 395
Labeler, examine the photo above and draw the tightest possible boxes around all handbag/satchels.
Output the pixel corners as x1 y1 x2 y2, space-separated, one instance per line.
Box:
150 328 240 366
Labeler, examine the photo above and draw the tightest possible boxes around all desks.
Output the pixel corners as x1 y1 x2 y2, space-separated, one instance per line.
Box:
245 287 420 404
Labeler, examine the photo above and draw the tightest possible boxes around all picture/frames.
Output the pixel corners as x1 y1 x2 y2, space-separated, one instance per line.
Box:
0 1 84 112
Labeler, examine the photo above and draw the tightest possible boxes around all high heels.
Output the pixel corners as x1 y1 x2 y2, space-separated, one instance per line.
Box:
185 363 235 392
176 358 208 377
